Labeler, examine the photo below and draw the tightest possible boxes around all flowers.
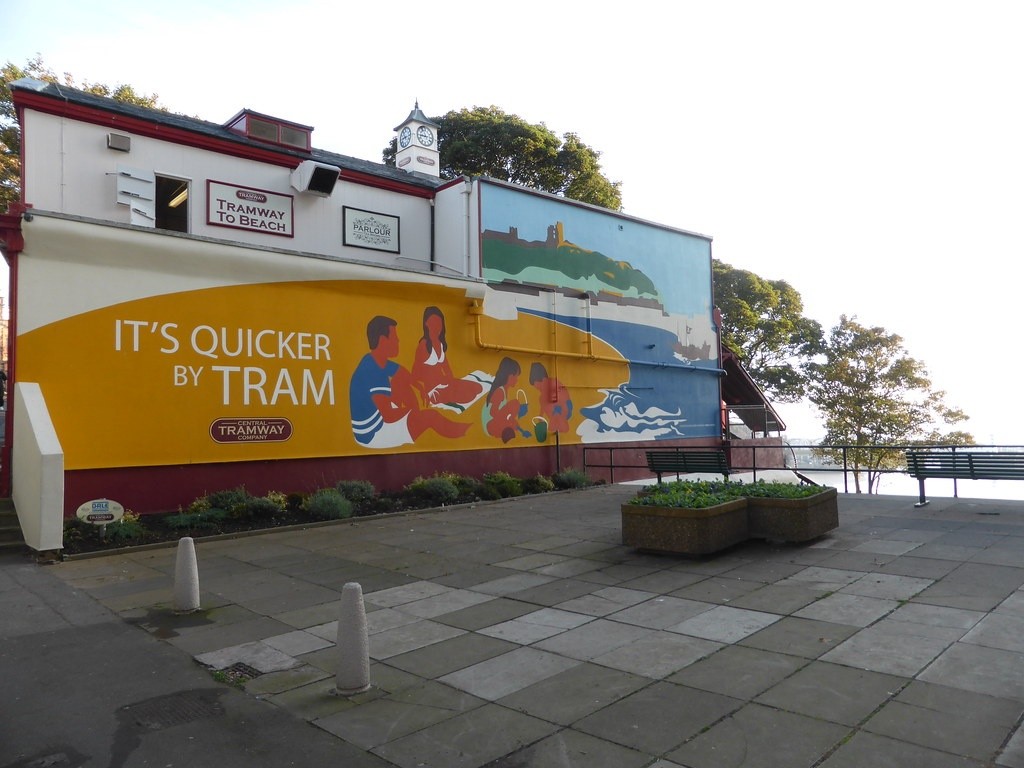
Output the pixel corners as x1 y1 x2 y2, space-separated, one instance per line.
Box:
626 478 828 508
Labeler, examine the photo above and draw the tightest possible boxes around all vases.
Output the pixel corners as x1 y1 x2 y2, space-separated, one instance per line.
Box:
620 486 840 554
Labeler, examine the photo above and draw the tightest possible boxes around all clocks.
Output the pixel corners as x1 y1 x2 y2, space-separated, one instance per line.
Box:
417 125 434 147
400 126 411 148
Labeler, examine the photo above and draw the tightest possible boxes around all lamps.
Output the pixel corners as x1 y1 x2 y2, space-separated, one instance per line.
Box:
290 160 341 198
108 133 130 152
167 183 188 209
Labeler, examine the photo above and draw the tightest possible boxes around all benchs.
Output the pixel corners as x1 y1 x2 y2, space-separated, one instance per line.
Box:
645 451 740 483
905 451 1024 508
